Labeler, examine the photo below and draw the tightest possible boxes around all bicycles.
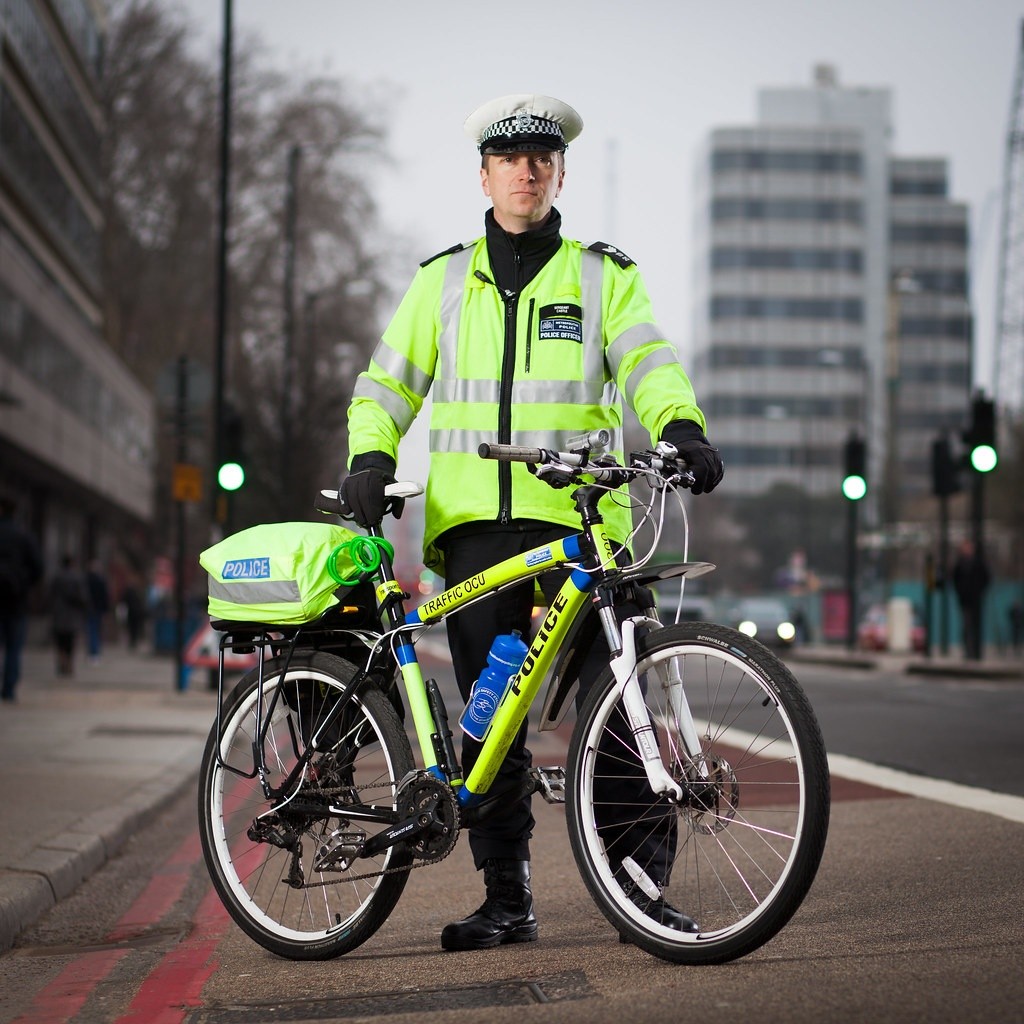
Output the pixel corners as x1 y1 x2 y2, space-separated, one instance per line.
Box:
195 428 830 963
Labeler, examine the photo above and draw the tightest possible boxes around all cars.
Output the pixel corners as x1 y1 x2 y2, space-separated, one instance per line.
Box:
858 603 924 650
731 601 795 651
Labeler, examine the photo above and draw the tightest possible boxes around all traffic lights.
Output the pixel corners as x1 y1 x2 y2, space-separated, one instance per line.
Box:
969 398 998 474
842 440 868 499
218 416 247 491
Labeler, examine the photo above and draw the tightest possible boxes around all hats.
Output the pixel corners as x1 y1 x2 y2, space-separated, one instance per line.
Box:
462 93 585 154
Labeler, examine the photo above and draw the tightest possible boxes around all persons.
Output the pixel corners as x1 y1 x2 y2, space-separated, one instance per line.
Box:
85 555 110 664
123 576 148 650
46 557 86 679
785 555 819 645
953 543 991 660
342 95 725 950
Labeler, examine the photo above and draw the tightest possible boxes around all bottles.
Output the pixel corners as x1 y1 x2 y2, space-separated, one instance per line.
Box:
458 629 532 741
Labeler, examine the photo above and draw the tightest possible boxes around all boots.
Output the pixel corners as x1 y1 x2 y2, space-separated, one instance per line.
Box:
440 859 538 951
618 881 700 945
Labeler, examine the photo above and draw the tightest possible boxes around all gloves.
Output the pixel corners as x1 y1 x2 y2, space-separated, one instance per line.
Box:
338 450 405 529
658 419 725 496
